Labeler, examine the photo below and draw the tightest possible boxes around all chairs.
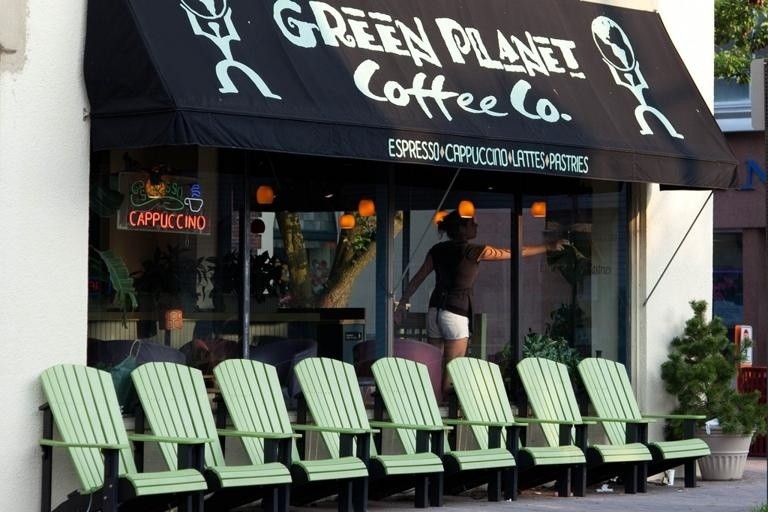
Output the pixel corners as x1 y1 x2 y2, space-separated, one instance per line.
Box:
89 336 114 371
257 339 319 399
353 338 443 412
130 361 292 510
517 356 647 498
179 336 258 413
576 356 712 493
213 357 368 511
371 356 515 508
445 356 584 502
104 337 187 412
40 363 206 510
293 356 442 511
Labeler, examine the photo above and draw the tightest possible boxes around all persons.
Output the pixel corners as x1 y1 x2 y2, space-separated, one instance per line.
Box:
390 205 577 396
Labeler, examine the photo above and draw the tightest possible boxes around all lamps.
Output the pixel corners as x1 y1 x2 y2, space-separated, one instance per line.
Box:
256 185 545 228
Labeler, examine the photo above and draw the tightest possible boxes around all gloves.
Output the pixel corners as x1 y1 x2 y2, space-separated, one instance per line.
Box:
547 237 571 252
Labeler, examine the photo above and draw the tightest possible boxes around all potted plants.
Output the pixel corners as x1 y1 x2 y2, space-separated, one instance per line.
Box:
658 298 766 482
